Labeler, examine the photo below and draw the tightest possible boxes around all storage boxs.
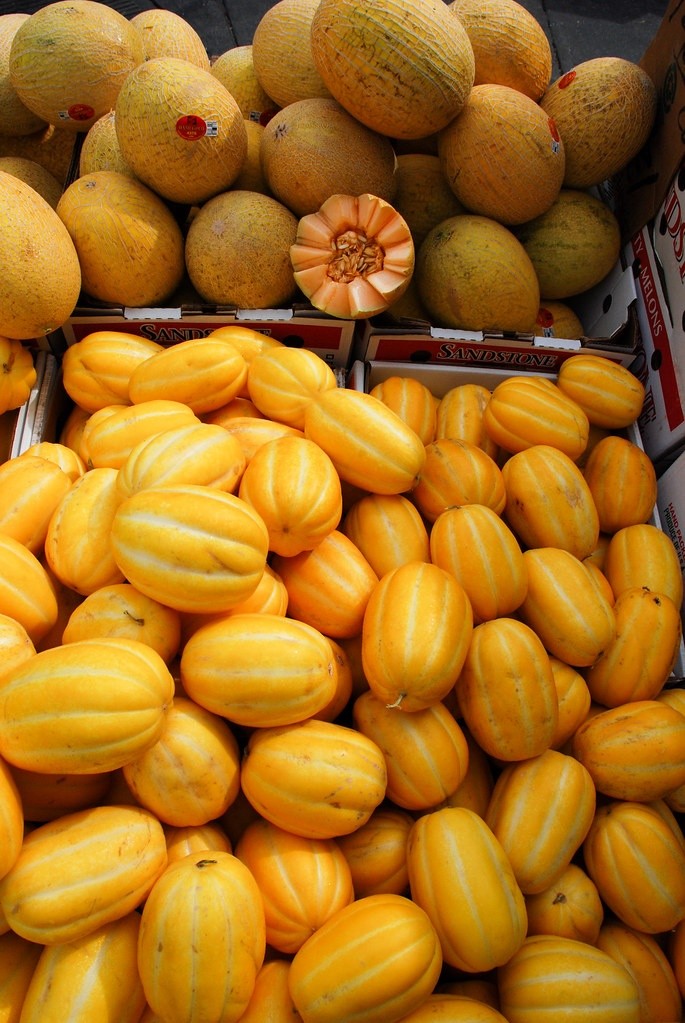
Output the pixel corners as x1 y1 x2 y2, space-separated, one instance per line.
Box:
1 160 685 689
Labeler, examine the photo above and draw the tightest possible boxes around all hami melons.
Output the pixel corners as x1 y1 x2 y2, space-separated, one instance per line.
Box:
1 0 658 341
